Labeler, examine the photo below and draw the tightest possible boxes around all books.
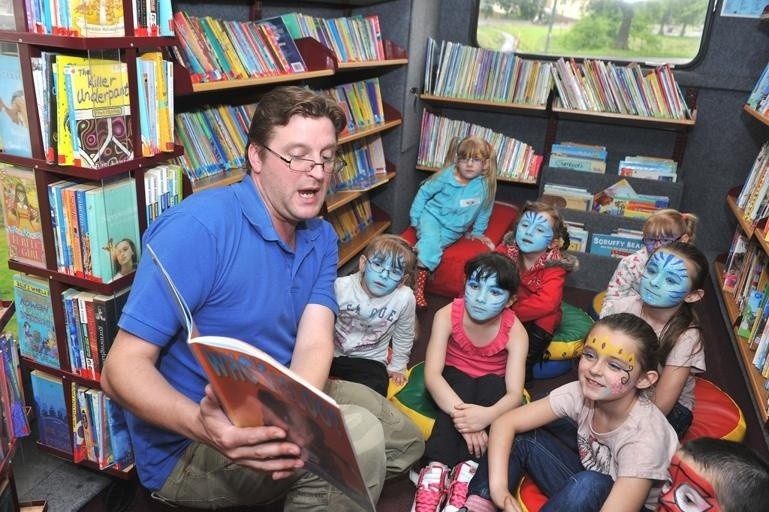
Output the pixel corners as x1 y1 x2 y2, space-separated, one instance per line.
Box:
723 60 769 409
418 108 542 182
1 4 181 471
172 11 387 245
426 37 692 120
147 243 377 512
543 141 678 256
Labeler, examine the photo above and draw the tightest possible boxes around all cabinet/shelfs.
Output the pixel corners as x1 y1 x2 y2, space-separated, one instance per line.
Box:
0 301 48 512
164 1 409 268
415 83 699 292
714 65 769 435
0 1 184 480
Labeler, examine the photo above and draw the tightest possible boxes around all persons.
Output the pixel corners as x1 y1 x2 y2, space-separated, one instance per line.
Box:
602 208 700 310
493 200 580 387
413 254 529 511
406 138 497 308
653 436 769 511
100 86 425 512
600 242 708 438
334 234 417 397
457 312 680 512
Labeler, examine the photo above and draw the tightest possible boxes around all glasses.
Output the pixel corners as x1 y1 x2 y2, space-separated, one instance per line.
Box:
643 234 681 247
366 256 406 282
260 143 347 175
457 155 487 165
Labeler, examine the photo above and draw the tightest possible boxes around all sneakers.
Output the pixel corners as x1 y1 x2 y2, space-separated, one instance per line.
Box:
444 459 479 512
411 461 447 512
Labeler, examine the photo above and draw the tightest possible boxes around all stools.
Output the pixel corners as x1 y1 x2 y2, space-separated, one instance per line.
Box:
524 295 593 380
515 473 548 512
386 347 409 400
682 377 747 445
397 202 521 301
390 362 531 443
